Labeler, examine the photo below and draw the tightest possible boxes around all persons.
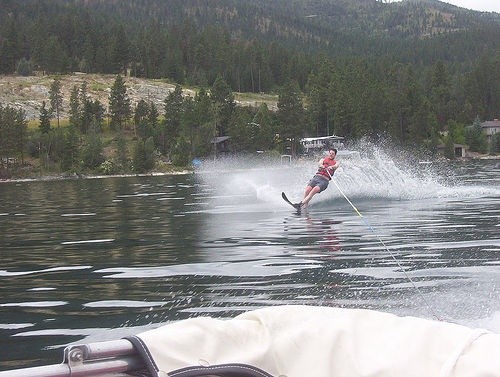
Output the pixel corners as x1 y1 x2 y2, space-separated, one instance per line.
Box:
293 147 342 215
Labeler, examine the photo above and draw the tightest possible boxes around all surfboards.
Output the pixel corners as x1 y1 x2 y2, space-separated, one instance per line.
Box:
282 193 303 210
